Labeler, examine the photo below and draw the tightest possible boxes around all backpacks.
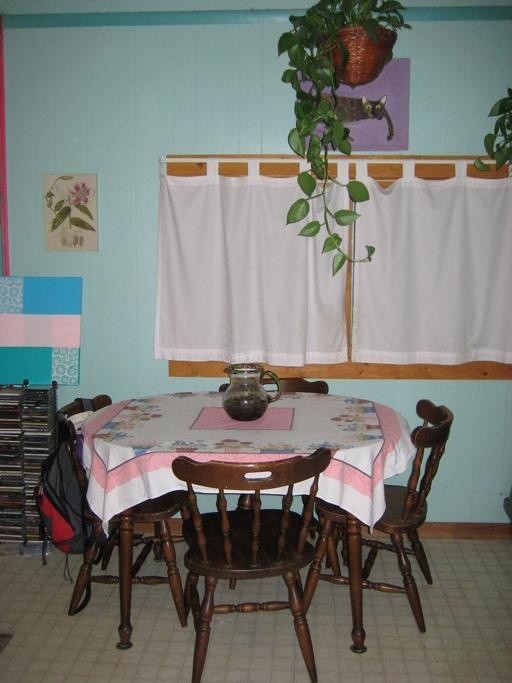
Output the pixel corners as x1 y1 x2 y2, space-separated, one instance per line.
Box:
34 412 94 585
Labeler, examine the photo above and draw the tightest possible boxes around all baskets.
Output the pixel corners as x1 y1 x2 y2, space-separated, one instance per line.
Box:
311 24 396 85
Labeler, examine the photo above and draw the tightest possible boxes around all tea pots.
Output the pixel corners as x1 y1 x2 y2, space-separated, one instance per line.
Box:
222 363 282 422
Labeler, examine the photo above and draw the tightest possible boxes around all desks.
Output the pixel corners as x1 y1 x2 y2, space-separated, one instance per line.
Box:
81 389 418 654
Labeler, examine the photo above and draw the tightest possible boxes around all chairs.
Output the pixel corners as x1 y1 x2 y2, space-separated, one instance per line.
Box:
55 394 188 650
302 399 454 632
171 446 332 683
218 377 342 590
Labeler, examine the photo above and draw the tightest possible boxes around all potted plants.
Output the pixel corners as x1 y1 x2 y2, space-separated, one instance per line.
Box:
277 0 414 278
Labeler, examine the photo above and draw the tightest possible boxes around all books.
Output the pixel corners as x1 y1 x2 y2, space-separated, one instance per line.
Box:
0 384 55 542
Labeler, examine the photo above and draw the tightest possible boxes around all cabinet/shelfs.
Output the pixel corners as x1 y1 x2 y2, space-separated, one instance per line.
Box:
0 379 59 565
